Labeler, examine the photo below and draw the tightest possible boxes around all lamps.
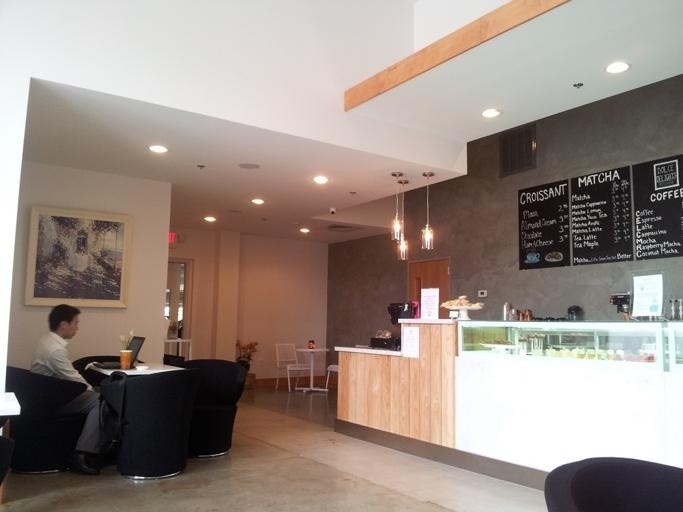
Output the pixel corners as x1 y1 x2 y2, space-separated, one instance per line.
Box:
390 172 436 262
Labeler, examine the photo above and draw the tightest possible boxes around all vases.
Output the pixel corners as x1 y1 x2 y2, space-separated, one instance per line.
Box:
237 359 250 372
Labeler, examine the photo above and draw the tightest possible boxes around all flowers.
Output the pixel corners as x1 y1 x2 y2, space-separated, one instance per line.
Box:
118 329 136 350
235 338 259 361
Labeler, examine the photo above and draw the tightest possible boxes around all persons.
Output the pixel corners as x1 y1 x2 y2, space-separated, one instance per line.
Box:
27 304 109 475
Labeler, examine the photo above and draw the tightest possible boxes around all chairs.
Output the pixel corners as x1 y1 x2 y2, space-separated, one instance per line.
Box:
543 457 683 511
6 353 246 483
275 344 339 394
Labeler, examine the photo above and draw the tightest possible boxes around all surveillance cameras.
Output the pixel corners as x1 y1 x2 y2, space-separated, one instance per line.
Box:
330 208 335 215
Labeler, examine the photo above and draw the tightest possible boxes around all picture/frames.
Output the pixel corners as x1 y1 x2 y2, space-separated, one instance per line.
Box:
23 205 132 308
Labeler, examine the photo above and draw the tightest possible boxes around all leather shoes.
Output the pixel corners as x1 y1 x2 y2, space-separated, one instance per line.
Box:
71 454 100 475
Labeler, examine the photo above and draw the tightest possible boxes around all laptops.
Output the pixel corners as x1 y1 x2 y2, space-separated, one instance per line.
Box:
93 336 146 369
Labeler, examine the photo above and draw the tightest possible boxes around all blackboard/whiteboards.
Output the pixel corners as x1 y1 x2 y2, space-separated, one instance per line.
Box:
519 152 683 270
